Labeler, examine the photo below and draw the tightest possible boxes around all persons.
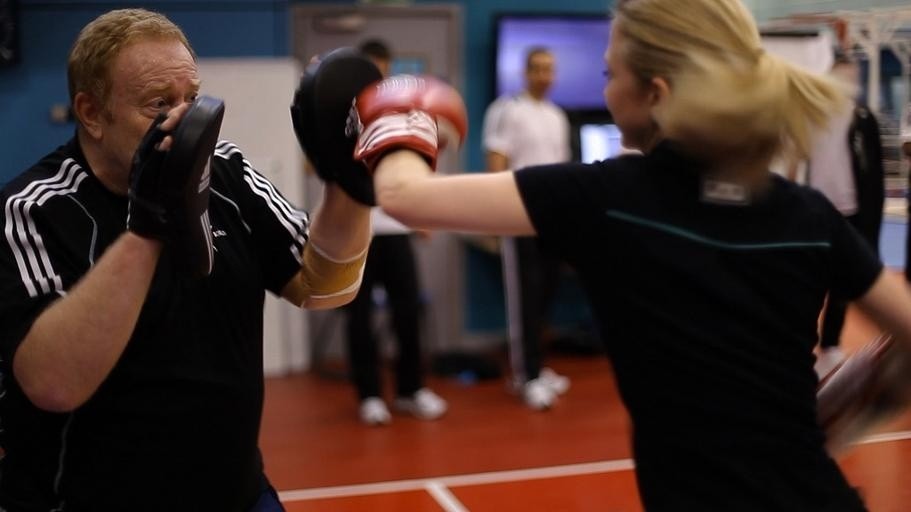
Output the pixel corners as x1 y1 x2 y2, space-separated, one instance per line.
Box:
2 9 384 512
345 0 911 512
348 38 445 423
482 47 569 407
795 58 885 374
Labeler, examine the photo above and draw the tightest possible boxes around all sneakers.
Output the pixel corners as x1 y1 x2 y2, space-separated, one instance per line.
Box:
507 366 570 411
359 396 392 429
394 387 446 422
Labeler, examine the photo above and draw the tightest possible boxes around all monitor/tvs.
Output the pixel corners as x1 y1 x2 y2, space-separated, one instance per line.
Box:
580 125 622 164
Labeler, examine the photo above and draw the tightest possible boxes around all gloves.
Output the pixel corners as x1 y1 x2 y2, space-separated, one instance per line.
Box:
353 72 469 170
819 337 886 448
